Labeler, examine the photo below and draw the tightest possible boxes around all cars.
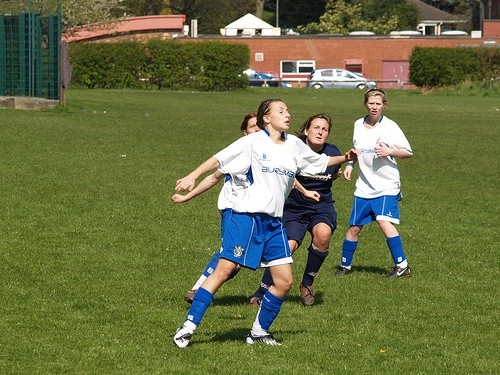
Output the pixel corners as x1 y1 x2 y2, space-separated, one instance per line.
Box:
306 68 377 90
239 68 293 88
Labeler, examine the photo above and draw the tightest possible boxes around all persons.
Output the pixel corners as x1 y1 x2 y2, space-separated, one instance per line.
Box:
164 97 362 347
334 88 413 279
249 113 343 306
174 110 321 306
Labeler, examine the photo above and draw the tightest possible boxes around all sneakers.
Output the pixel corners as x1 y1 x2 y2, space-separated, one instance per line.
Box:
387 264 412 279
174 324 193 349
300 282 315 306
246 329 282 346
335 265 353 276
250 296 261 306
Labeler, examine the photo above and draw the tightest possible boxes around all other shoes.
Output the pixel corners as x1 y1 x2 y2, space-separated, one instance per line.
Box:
184 289 198 303
